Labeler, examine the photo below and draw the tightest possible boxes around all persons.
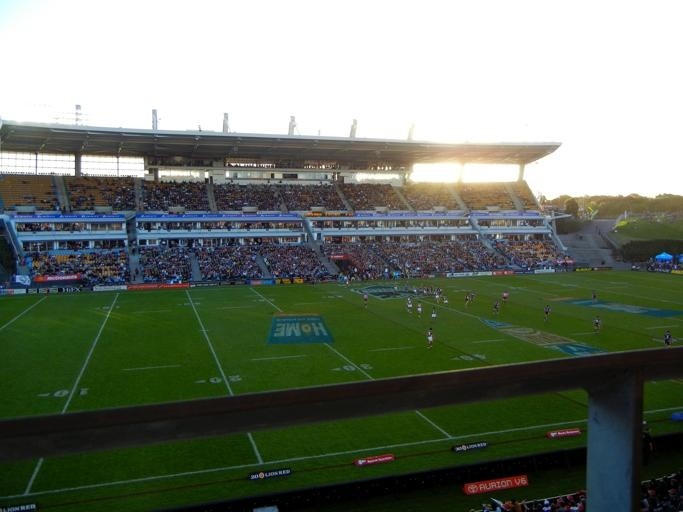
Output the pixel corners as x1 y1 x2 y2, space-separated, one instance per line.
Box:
364 284 448 321
464 290 509 312
0 171 569 283
544 305 550 320
664 330 672 344
592 289 600 331
452 467 683 511
631 252 679 274
427 328 433 347
